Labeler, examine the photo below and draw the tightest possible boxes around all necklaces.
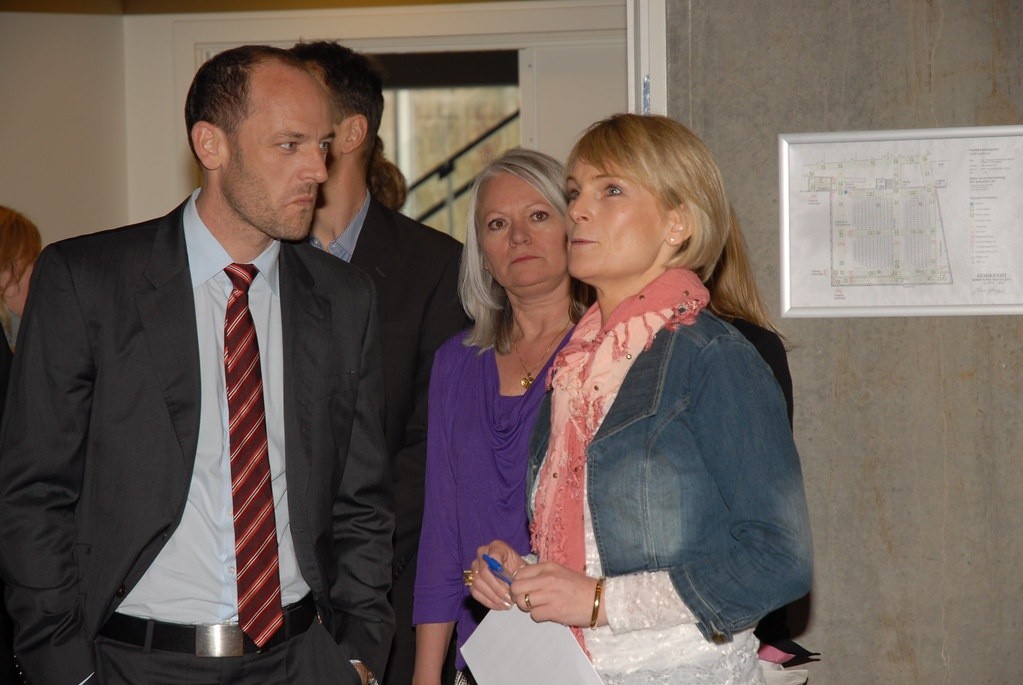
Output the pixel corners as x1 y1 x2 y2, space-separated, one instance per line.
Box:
512 320 571 390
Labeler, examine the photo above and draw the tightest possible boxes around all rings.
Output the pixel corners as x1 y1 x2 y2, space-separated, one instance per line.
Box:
463 570 479 586
525 593 532 608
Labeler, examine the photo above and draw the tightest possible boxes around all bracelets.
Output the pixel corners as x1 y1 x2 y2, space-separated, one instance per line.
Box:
590 576 603 627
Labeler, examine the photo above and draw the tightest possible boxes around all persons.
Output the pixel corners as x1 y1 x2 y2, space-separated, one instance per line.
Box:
410 113 821 685
0 39 475 685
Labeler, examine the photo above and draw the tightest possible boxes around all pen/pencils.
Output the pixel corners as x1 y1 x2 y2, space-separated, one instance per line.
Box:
482 554 516 586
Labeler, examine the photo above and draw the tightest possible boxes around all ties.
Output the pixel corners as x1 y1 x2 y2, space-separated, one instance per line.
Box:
222 258 282 646
